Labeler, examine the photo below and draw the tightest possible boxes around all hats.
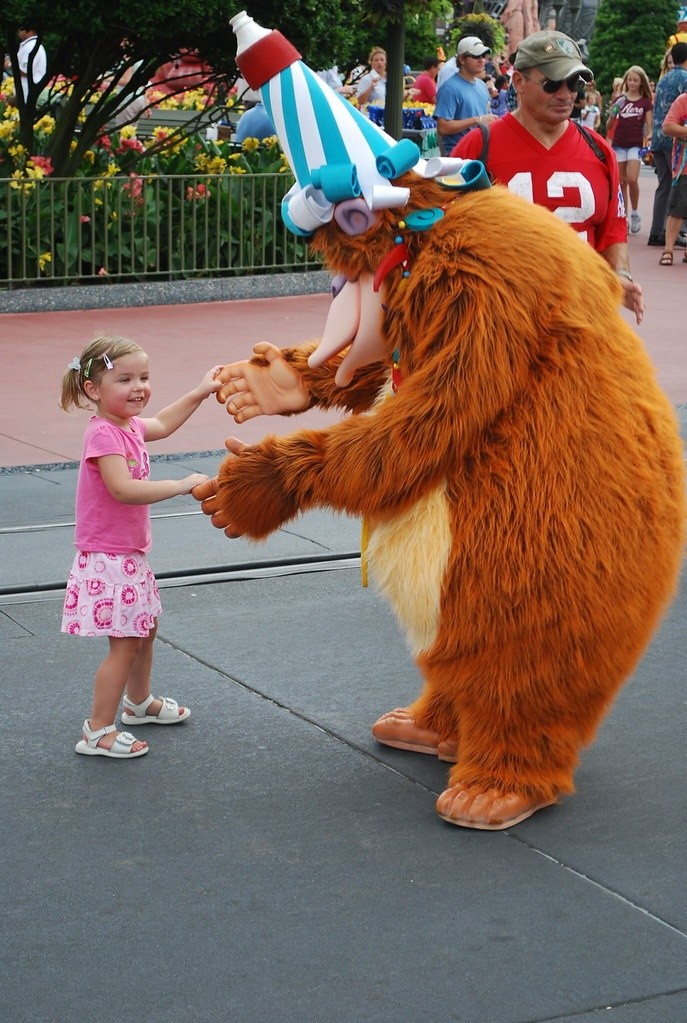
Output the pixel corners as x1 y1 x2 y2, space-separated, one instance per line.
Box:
457 36 492 56
514 30 594 82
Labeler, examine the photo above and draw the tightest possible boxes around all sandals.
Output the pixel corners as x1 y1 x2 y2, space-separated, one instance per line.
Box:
120 695 190 725
76 719 150 760
660 249 673 265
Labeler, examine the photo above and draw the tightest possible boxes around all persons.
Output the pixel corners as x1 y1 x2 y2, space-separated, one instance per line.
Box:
115 81 152 134
449 29 645 325
3 19 49 110
333 36 687 266
58 336 226 757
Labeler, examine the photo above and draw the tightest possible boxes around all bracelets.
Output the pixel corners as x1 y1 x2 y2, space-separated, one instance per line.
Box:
618 270 633 281
479 115 482 123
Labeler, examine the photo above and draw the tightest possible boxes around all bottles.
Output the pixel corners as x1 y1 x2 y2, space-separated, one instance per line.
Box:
610 96 626 116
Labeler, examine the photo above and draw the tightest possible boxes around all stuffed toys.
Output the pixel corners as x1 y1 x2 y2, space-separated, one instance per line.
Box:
190 12 687 830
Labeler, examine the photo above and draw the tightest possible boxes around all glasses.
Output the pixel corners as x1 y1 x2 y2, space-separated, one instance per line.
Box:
464 54 486 59
519 70 585 95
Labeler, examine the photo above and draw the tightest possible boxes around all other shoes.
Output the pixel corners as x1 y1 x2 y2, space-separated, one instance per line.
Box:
677 235 687 245
648 236 665 246
630 215 641 233
